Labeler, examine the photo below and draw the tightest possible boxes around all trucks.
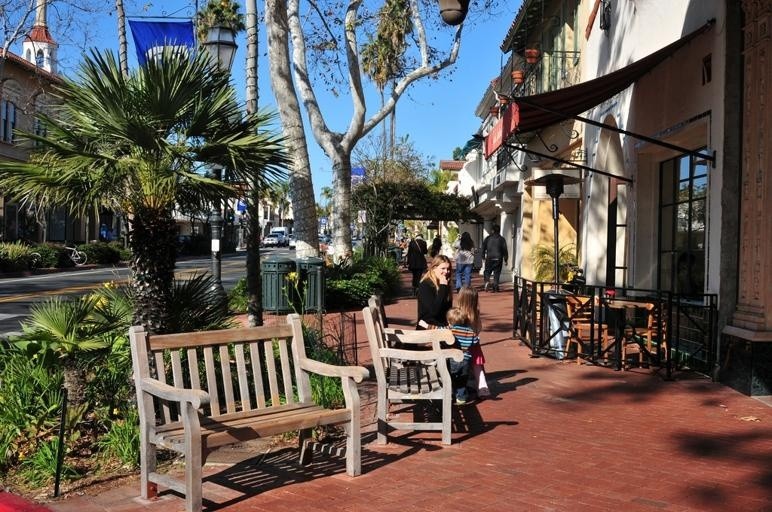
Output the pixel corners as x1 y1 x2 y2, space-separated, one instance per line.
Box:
270 226 289 247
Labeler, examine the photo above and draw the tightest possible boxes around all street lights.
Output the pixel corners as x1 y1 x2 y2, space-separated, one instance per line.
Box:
202 28 238 316
524 174 585 294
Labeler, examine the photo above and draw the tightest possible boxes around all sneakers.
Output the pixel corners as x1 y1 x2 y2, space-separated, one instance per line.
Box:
478 388 490 398
456 398 466 404
484 282 490 292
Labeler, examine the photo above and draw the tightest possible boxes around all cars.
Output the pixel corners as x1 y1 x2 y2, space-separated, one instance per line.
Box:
317 233 361 263
263 233 285 248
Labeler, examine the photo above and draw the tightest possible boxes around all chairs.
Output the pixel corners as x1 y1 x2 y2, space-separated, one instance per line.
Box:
527 284 668 372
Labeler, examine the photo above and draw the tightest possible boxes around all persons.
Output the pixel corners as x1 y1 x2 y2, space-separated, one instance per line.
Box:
419 306 480 407
456 283 492 400
101 219 115 243
452 231 476 293
432 234 443 255
414 254 455 330
407 233 429 287
481 224 508 293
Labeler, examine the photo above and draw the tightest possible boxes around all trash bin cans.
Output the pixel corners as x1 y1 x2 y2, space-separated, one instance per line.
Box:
261 255 326 316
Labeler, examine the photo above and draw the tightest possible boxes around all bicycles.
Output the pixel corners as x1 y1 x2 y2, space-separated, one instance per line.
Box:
54 242 87 265
